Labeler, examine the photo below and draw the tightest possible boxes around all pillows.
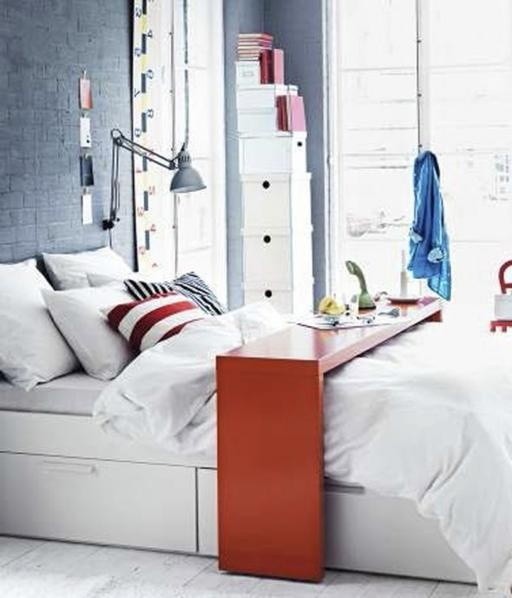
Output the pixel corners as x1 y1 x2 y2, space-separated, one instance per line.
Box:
37 277 148 384
0 251 85 396
42 243 166 289
80 267 137 291
493 293 512 320
97 283 214 350
124 266 233 318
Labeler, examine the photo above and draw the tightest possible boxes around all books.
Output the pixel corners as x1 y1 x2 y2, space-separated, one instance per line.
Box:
237 32 307 132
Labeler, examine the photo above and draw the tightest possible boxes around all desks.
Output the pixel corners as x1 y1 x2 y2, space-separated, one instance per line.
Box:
210 291 446 587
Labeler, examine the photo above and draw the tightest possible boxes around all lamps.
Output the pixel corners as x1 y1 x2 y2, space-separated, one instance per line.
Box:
98 125 207 233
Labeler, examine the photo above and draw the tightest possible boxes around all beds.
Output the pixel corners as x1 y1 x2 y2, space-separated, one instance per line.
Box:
0 297 512 597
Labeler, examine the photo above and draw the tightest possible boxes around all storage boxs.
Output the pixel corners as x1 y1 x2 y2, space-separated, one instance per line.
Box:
231 56 316 317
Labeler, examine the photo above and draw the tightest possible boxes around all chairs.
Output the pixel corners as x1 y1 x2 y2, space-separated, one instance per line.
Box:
486 255 512 335
